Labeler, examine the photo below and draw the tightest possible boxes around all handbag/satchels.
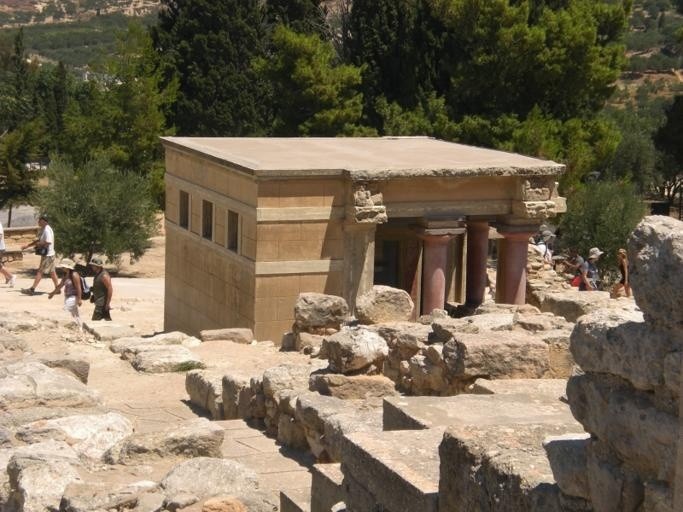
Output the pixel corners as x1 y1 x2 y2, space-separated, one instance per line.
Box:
571 275 582 286
79 277 90 300
34 246 48 256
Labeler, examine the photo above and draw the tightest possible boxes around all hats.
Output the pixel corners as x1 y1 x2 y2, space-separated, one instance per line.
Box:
588 247 604 259
88 258 105 268
56 258 76 270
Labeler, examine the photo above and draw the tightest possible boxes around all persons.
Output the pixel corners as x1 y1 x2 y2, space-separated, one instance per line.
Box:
579 247 604 291
612 248 631 297
89 257 112 321
18 215 59 295
47 257 81 317
0 222 16 289
563 248 584 287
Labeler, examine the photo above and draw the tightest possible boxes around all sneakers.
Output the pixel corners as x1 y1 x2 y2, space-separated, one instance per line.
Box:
9 275 16 287
21 287 34 296
5 278 9 284
